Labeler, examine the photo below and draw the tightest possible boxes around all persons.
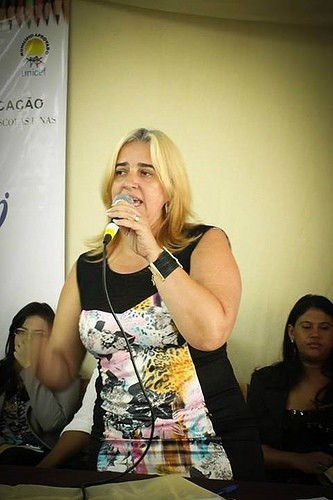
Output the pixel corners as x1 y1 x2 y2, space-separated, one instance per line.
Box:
34 124 270 484
0 300 106 470
247 293 333 487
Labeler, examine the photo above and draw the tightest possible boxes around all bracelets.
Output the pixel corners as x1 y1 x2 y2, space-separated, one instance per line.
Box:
147 244 184 280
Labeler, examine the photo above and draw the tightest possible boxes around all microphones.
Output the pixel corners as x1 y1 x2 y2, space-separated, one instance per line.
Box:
103 193 133 248
